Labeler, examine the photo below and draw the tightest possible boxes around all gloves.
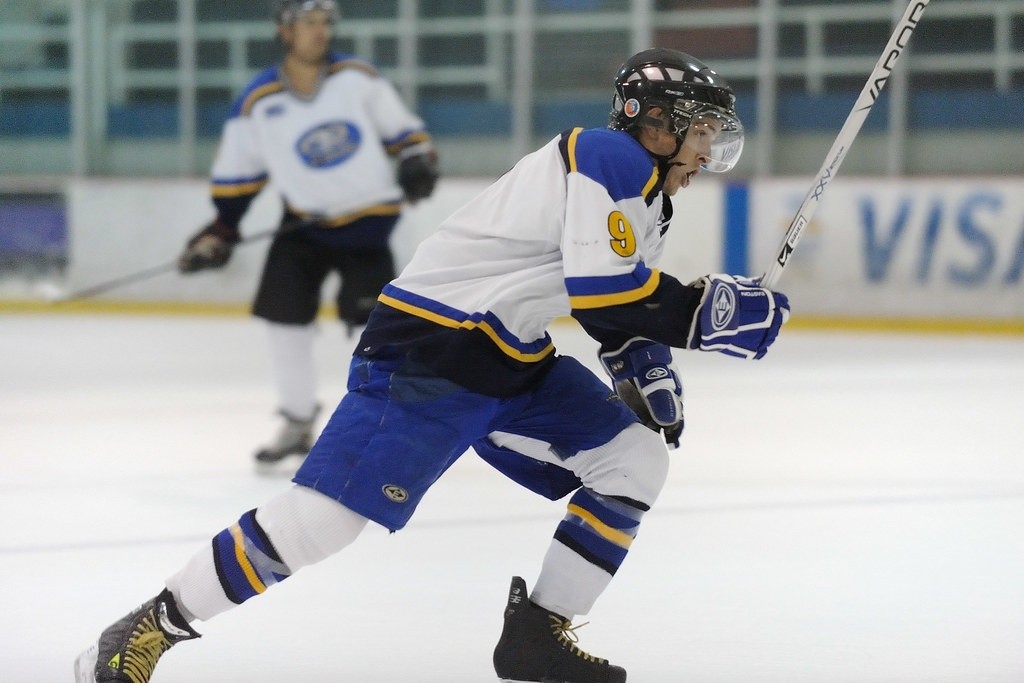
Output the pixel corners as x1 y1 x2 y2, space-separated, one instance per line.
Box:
386 131 436 200
682 273 792 362
179 220 241 273
597 336 685 449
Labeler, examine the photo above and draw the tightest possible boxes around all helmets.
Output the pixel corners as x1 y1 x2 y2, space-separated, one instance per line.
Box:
610 47 741 139
274 0 341 26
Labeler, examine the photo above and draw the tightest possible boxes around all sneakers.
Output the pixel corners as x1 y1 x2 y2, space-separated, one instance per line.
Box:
493 575 627 682
71 586 201 682
254 405 317 474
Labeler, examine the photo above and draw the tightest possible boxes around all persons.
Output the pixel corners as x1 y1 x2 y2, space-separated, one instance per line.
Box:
74 49 791 683
175 0 442 476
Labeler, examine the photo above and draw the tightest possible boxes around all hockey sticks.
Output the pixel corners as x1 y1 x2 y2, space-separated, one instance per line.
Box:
26 227 274 308
749 0 928 293
